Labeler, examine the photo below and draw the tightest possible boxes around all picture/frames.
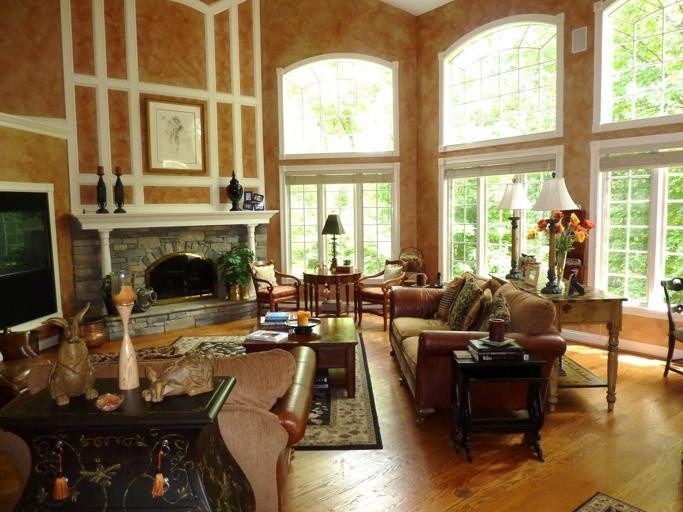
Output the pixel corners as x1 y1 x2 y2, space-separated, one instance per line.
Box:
243 190 264 211
144 98 207 175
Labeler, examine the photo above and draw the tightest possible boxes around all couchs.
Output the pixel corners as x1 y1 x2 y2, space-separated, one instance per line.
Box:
0 346 318 512
389 286 566 427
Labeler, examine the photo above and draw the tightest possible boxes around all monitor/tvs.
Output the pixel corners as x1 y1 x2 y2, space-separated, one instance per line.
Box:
0 207 49 282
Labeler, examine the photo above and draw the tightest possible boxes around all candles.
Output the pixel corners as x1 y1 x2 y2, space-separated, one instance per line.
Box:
97 166 104 174
116 166 121 173
297 310 309 324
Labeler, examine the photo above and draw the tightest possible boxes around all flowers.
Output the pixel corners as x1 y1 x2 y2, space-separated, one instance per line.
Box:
527 212 595 252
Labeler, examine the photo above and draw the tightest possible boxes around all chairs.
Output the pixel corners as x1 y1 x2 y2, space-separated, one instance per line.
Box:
356 260 408 331
247 260 301 312
399 247 426 286
661 278 683 378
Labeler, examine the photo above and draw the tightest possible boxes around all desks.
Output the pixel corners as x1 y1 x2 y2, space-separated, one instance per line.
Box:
449 353 548 464
489 273 628 412
303 270 361 318
0 376 256 512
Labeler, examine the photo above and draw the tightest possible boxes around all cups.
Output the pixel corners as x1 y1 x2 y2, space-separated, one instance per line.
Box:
487 318 505 342
417 272 428 286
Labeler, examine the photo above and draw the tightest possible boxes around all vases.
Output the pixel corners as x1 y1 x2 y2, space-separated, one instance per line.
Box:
226 170 243 211
555 250 567 288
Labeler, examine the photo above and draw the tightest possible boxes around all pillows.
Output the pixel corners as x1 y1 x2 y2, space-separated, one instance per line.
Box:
438 272 556 335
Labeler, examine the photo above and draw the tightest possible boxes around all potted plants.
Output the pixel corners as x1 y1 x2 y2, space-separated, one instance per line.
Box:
217 245 255 301
100 272 120 316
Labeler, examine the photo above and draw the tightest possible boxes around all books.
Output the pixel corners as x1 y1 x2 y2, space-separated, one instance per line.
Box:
465 335 531 362
258 310 293 326
313 369 330 383
311 382 330 389
246 329 290 343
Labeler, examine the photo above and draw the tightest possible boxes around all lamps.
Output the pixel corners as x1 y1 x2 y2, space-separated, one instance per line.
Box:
322 215 344 271
532 173 580 294
496 178 533 278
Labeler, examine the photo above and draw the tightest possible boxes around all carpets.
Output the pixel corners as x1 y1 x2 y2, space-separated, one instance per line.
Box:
573 492 646 512
169 333 383 450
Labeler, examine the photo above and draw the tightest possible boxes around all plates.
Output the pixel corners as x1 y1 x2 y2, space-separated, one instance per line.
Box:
285 319 322 328
